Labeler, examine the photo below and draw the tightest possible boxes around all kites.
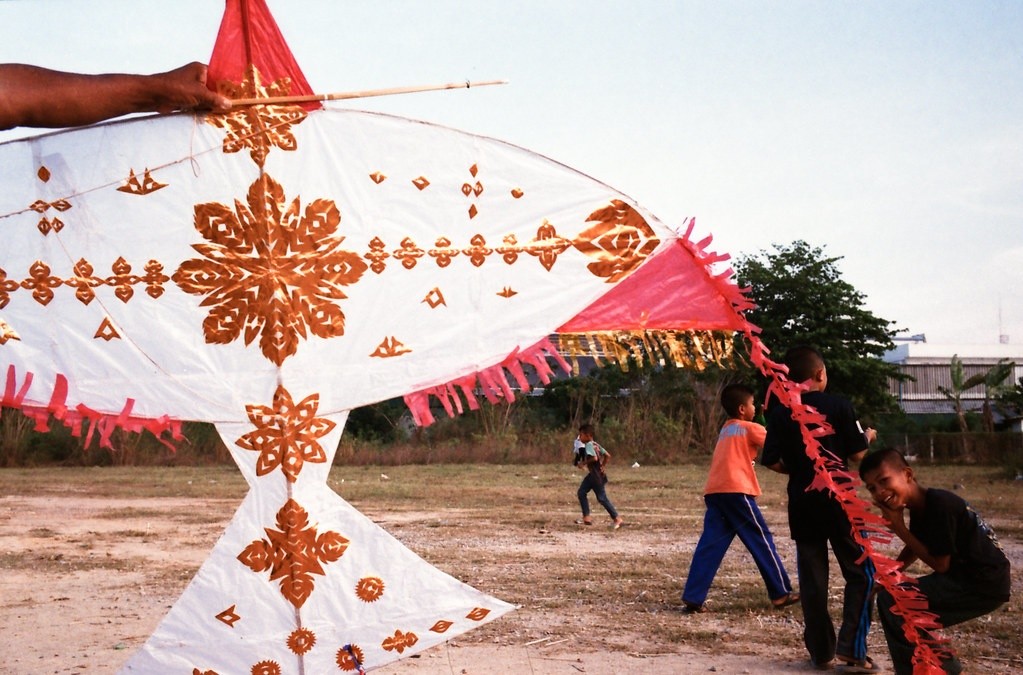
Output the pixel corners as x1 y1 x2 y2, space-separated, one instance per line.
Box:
0 0 957 675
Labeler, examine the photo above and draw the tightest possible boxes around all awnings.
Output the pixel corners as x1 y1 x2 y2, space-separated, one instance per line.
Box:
900 397 1003 415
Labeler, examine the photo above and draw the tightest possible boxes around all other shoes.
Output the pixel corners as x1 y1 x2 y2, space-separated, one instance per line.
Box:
574 518 592 525
610 521 625 530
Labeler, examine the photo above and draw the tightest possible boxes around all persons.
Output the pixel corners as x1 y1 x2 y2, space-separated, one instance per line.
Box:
574 423 625 529
858 445 1011 675
572 434 586 467
760 345 882 673
680 382 801 613
0 60 233 132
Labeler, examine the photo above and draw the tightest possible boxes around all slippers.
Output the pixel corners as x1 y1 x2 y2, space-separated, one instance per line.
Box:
834 656 885 673
775 591 800 609
811 656 838 670
681 604 709 614
